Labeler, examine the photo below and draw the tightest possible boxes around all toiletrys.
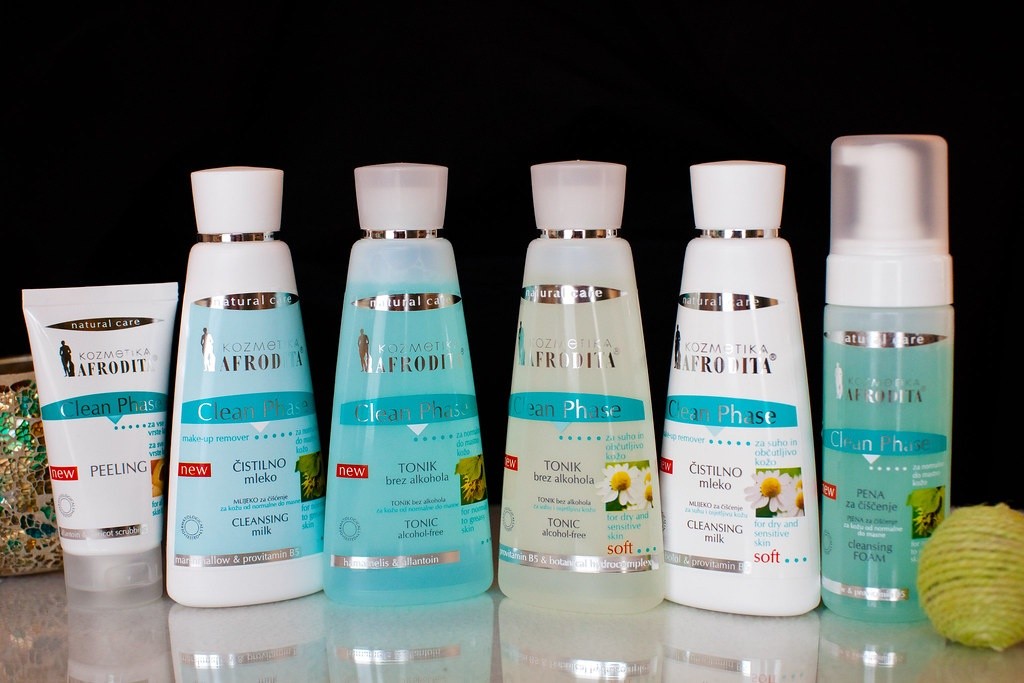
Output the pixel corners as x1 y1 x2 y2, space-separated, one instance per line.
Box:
17 127 961 620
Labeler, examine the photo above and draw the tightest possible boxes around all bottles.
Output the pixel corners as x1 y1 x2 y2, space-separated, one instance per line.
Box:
322 161 494 606
498 160 667 614
659 159 822 619
165 167 328 609
